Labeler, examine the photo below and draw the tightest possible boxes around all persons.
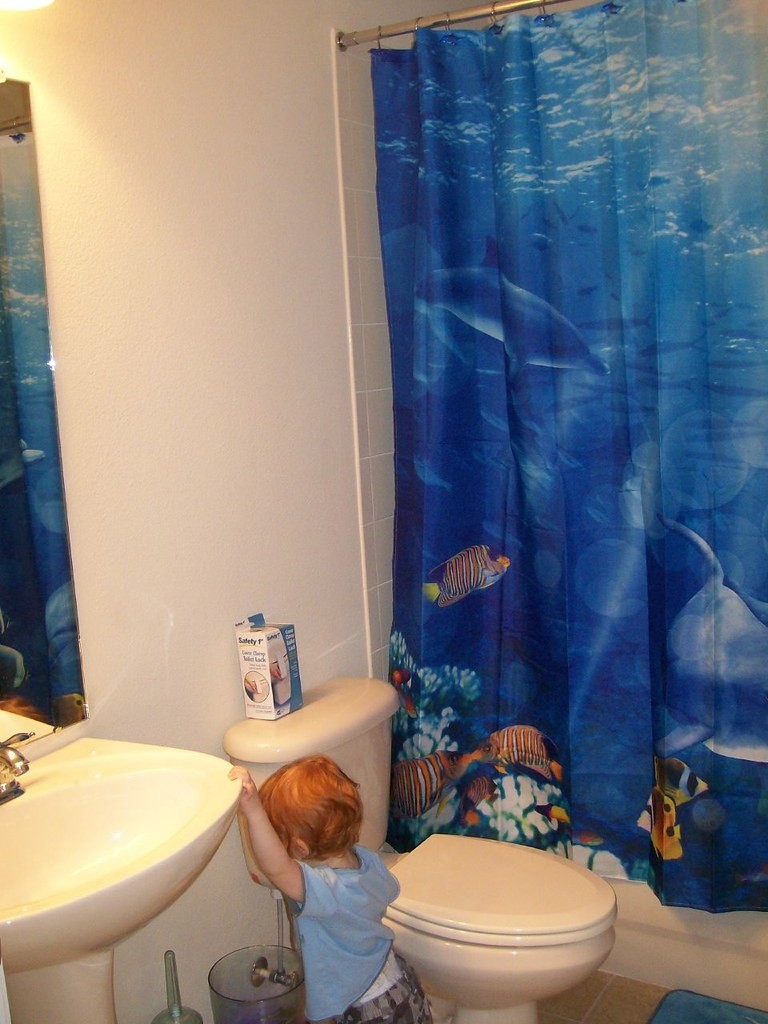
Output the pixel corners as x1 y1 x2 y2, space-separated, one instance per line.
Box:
229 756 434 1024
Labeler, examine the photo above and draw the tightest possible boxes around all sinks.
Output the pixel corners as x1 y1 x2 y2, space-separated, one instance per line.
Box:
0 734 244 979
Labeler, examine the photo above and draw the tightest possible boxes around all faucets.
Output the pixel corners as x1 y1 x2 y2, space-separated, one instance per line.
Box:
0 731 36 805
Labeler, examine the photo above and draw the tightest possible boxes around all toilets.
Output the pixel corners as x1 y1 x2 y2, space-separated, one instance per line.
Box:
221 676 619 1023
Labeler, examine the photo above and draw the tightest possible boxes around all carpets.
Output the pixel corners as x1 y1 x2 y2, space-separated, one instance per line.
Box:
646 987 768 1024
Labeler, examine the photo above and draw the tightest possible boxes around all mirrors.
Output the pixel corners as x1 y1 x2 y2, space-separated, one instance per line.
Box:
0 79 91 750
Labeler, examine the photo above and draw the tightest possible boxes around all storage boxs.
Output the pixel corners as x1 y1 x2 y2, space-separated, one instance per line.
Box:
234 613 303 721
